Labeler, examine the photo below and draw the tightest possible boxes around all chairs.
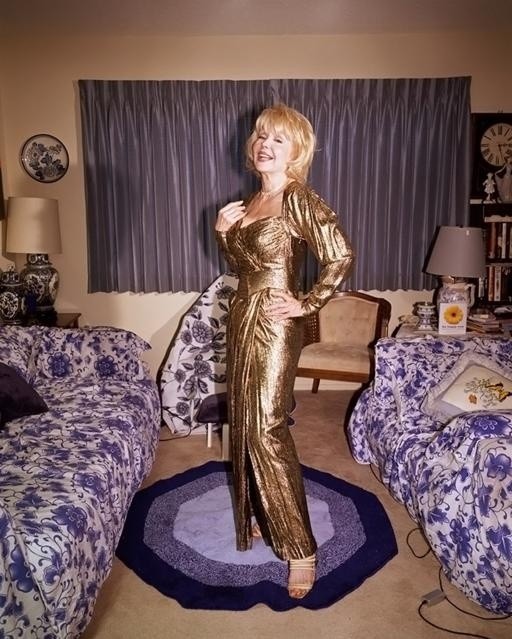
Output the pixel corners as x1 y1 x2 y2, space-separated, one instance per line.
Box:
345 335 511 615
293 290 391 393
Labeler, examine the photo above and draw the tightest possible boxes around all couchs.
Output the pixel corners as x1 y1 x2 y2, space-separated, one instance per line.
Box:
0 323 162 639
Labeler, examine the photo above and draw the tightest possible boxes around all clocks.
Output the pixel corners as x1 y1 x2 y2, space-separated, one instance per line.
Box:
469 112 511 200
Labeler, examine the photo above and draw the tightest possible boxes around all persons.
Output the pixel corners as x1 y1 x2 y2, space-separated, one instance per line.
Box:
215 108 355 600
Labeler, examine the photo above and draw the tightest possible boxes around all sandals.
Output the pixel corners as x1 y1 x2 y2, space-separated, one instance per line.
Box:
251 515 261 536
288 553 316 599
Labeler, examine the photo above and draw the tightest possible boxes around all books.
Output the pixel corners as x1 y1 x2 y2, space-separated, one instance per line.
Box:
467 315 504 334
478 216 512 302
438 302 467 335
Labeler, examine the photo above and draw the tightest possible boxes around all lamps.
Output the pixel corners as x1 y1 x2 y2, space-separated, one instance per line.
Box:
7 195 64 312
423 225 488 316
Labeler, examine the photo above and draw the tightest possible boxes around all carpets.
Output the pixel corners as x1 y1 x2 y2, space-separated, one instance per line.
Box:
113 458 399 612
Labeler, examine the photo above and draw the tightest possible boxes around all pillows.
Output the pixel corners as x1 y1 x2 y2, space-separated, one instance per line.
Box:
418 348 511 425
0 361 50 424
